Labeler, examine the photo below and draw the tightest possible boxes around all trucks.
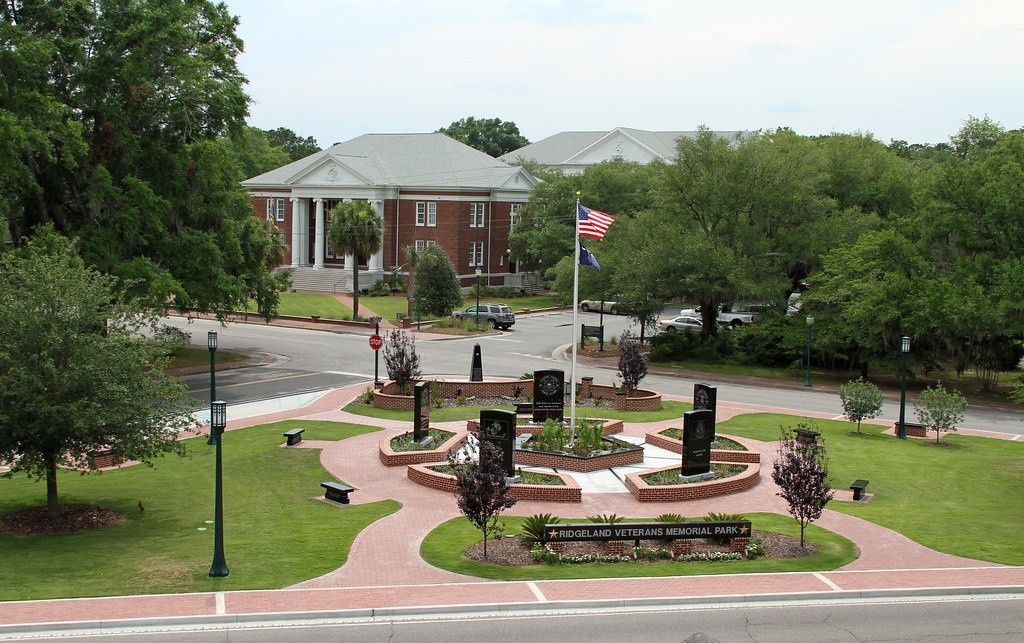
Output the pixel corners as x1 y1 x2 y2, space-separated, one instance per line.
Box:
680 301 755 330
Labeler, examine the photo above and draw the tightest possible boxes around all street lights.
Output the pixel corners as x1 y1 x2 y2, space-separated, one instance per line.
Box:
208 394 232 577
474 265 482 331
896 333 912 439
803 312 815 387
206 330 222 446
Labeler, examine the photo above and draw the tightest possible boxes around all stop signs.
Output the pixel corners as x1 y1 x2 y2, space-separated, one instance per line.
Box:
369 335 383 351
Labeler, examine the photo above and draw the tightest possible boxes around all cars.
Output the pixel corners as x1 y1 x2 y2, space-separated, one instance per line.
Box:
658 316 706 334
578 293 637 316
730 304 772 323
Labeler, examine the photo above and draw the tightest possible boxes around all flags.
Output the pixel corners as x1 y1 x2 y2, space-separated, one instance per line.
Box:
578 203 615 239
580 242 600 272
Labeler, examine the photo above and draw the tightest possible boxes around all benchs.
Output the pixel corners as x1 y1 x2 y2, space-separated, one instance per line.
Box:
850 479 870 501
87 449 123 468
793 429 822 444
895 422 929 437
283 428 305 446
321 482 355 504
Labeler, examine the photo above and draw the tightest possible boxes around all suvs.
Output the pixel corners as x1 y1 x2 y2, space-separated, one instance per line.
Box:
451 303 515 330
